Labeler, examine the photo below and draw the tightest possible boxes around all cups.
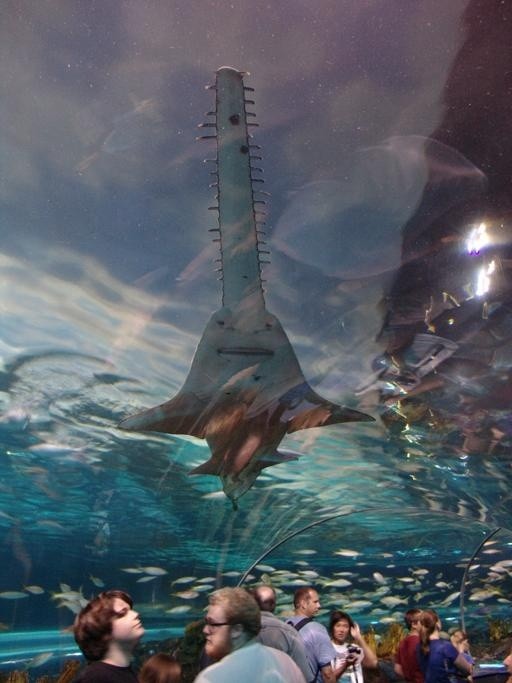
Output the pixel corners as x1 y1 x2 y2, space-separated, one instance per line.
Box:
201 616 245 631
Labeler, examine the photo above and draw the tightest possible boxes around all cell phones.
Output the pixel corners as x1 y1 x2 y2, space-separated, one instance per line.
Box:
346 643 361 654
